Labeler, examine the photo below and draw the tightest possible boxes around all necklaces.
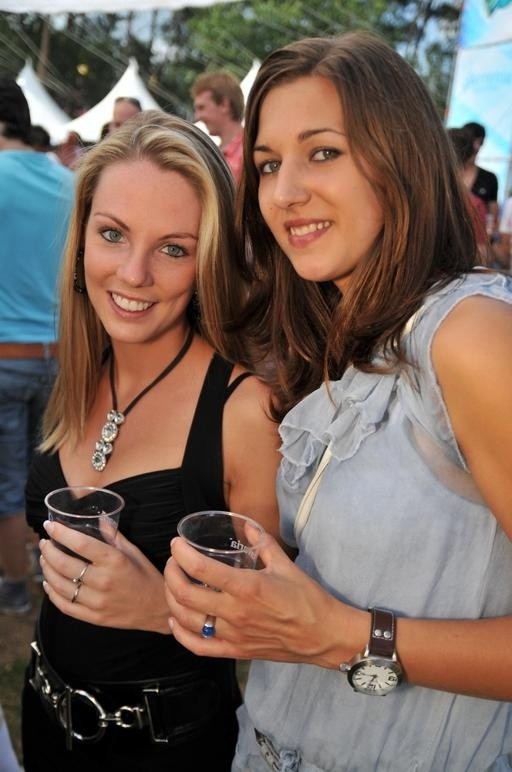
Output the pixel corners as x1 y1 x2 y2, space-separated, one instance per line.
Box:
91 330 195 472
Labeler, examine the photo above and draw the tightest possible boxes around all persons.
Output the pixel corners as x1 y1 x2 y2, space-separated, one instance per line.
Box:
108 96 142 132
31 122 63 166
0 73 81 619
20 104 288 772
160 28 512 772
192 67 246 188
448 120 511 274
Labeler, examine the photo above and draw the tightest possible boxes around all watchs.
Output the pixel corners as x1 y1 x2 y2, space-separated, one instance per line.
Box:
339 606 404 697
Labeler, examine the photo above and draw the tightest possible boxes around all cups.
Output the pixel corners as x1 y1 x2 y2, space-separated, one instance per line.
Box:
42 484 125 558
177 511 266 595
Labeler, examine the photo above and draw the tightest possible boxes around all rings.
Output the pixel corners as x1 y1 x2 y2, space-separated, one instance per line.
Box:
200 614 217 639
70 584 81 603
72 563 89 582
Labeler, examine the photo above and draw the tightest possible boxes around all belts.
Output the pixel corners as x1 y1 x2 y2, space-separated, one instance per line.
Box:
0 341 58 361
26 640 244 753
